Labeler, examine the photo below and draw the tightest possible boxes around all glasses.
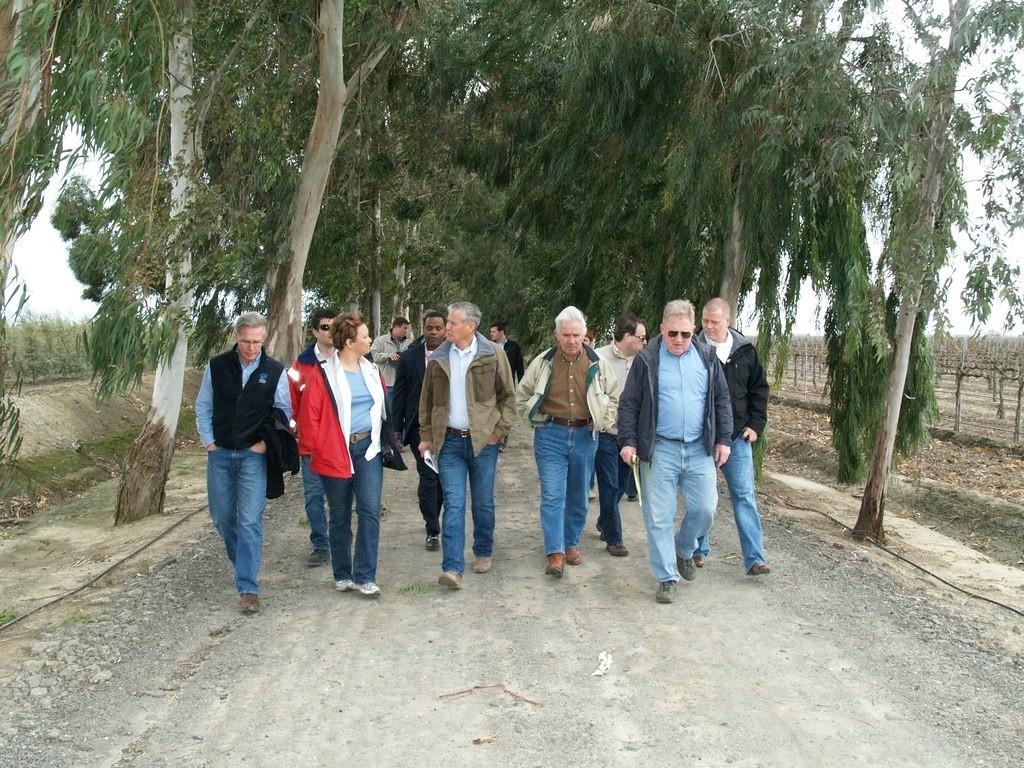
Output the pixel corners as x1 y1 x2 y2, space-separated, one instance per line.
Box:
316 324 331 331
663 327 693 338
238 335 265 346
629 333 647 342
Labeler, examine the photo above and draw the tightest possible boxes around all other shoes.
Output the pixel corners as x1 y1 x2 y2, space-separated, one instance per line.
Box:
437 571 462 590
307 549 330 566
748 564 770 576
241 594 260 611
588 489 595 499
474 555 491 573
628 495 637 502
694 556 704 567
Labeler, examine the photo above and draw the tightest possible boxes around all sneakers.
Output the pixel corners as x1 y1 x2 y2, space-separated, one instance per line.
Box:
677 556 696 580
656 579 678 602
336 579 352 591
352 582 380 596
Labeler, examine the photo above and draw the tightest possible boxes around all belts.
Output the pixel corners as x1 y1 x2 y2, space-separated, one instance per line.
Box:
350 431 372 443
548 417 588 428
447 428 470 437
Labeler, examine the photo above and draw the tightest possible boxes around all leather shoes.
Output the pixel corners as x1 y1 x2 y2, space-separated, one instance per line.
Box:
596 523 606 541
565 548 582 565
606 543 629 556
546 554 564 578
426 535 439 549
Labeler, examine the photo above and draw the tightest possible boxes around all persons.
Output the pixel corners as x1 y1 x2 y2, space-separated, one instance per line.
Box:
287 309 354 567
194 311 294 616
582 329 597 499
418 301 517 590
626 328 650 502
617 299 734 603
489 321 525 453
595 309 648 557
392 311 446 551
296 307 410 597
693 296 772 574
517 305 616 578
408 309 438 349
370 317 413 453
